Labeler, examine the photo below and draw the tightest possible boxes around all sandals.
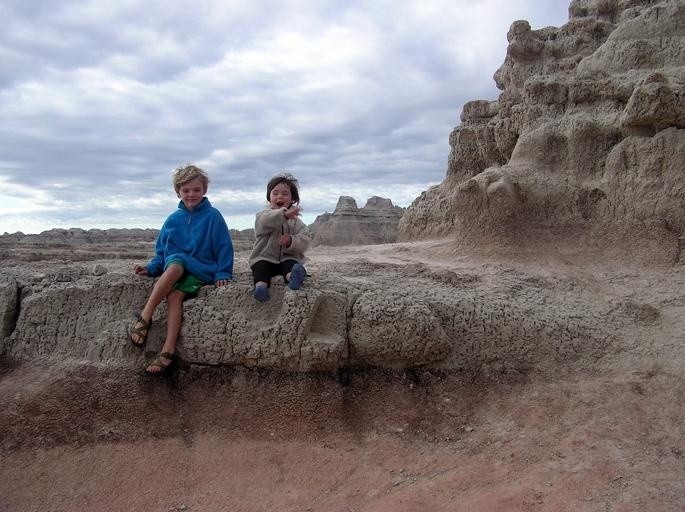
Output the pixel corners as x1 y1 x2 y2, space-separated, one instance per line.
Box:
131 314 152 346
145 348 178 375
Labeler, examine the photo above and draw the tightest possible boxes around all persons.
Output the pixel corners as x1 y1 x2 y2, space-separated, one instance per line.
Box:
130 166 233 375
249 171 311 302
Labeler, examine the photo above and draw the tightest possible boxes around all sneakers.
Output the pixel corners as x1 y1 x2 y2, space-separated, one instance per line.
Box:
289 263 306 290
255 286 269 301
